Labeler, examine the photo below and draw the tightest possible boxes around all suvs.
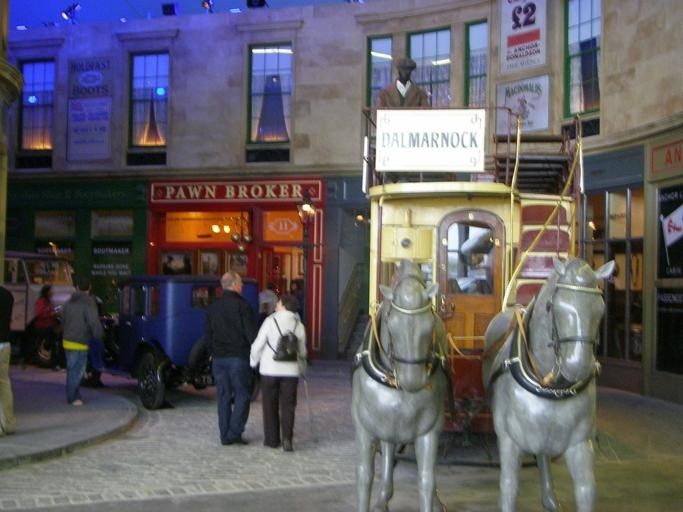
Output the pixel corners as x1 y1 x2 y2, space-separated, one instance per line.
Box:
4 248 77 367
77 277 262 410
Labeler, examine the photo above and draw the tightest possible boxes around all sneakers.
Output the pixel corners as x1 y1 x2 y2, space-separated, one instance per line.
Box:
224 433 294 451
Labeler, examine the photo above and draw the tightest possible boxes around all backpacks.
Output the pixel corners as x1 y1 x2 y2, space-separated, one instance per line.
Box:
268 318 299 361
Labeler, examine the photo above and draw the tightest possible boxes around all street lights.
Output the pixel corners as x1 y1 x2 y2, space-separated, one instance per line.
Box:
297 187 320 363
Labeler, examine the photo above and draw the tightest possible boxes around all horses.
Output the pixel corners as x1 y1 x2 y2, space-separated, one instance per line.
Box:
349 277 450 511
482 254 615 512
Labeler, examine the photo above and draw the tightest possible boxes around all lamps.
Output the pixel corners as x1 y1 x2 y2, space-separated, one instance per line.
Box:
199 0 214 15
300 188 313 212
352 207 366 224
229 206 254 254
59 3 82 27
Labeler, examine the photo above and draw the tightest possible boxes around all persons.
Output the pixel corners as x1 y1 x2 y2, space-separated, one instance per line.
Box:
289 280 305 321
58 277 108 407
377 56 430 109
0 284 18 437
85 296 114 389
258 283 280 326
248 292 310 452
203 270 260 447
18 283 62 371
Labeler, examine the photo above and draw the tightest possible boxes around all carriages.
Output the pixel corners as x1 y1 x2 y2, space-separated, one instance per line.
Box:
344 102 619 511
